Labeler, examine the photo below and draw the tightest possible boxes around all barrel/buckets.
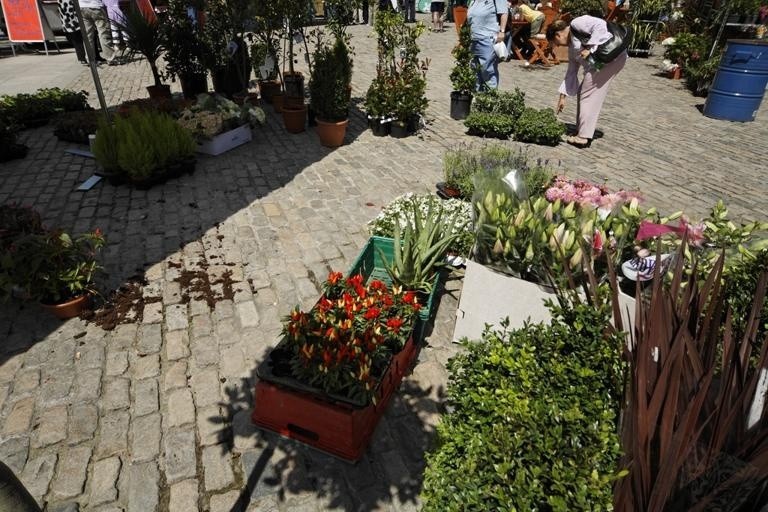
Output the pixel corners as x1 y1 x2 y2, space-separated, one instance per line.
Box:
702 39 768 123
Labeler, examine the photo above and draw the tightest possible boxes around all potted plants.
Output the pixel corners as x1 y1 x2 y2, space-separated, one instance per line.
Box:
448 14 485 120
363 2 432 138
97 0 354 149
449 59 480 121
11 221 115 319
463 86 569 146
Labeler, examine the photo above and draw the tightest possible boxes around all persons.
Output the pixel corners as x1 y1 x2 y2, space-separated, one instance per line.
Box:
430 1 445 34
544 12 628 148
464 0 511 97
366 0 380 26
515 1 548 40
352 0 366 26
55 0 89 65
103 1 132 51
77 0 126 66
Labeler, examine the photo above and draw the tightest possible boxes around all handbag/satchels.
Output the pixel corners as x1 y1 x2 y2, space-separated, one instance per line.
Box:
569 21 632 63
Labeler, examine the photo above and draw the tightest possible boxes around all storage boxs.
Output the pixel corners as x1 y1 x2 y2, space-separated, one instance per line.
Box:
450 260 586 344
609 283 768 428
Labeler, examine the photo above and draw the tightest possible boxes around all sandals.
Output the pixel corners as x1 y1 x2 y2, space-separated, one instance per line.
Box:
567 135 589 148
